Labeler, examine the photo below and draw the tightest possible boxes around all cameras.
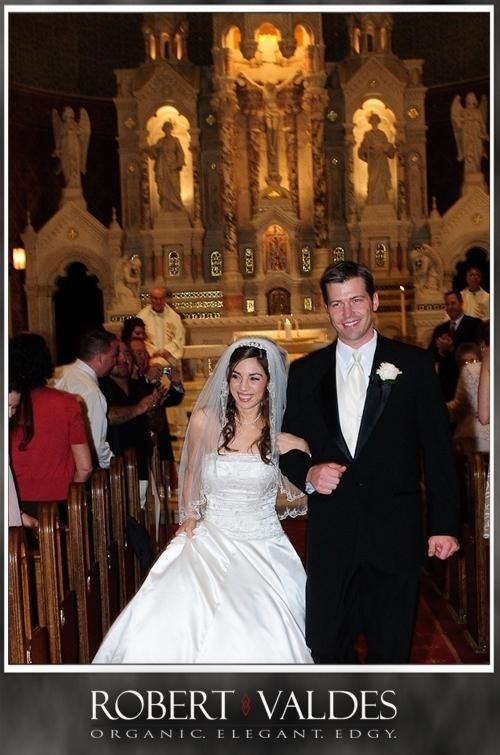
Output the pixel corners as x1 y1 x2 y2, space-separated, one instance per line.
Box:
163 368 171 374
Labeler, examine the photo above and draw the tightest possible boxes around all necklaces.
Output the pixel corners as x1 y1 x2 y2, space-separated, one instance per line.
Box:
234 412 261 424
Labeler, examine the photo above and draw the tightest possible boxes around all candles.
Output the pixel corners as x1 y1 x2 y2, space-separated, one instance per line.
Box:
399 284 406 338
283 317 294 341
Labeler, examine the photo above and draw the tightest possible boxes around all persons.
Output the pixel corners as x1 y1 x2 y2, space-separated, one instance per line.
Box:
50 105 91 189
451 92 488 172
237 69 303 155
89 332 318 665
427 264 490 545
146 121 185 211
357 112 395 204
277 260 467 665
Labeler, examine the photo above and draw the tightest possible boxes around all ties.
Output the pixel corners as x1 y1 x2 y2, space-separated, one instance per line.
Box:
450 322 457 334
341 351 369 458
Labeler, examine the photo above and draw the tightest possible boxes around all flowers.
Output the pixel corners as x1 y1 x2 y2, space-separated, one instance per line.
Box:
376 362 402 381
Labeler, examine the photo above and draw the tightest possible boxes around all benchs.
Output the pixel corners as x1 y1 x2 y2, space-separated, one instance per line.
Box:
428 447 490 658
9 440 180 664
